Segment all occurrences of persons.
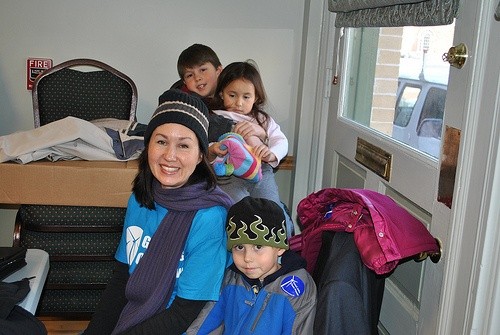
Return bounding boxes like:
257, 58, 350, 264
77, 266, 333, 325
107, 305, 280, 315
207, 59, 294, 239
182, 196, 317, 335
82, 89, 235, 335
170, 44, 223, 106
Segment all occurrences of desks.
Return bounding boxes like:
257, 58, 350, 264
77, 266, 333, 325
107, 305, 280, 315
0, 247, 49, 335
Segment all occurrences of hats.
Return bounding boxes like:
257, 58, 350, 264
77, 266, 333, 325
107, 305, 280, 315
225, 196, 289, 251
213, 132, 263, 184
144, 89, 210, 155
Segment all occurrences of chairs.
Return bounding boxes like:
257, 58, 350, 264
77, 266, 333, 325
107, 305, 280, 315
13, 59, 140, 321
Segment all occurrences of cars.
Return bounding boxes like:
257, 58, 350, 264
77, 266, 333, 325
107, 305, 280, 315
393, 75, 449, 159
